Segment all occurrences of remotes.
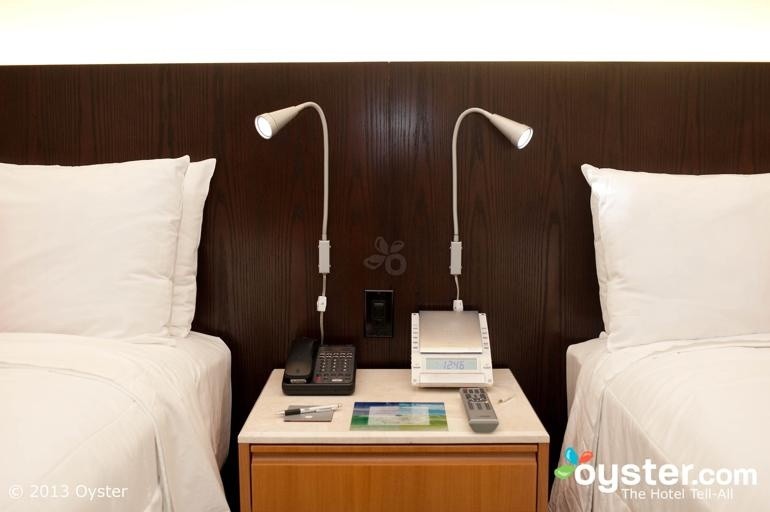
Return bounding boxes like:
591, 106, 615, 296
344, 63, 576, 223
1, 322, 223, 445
460, 387, 500, 433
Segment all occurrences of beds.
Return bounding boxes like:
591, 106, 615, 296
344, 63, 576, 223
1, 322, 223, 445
566, 338, 770, 512
1, 331, 232, 512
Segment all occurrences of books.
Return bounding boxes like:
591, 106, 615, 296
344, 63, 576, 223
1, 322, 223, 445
347, 400, 449, 434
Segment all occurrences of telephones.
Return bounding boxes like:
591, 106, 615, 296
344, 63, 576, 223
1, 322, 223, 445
282, 335, 357, 396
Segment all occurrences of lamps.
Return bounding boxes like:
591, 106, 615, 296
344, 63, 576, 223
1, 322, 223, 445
252, 101, 329, 344
450, 108, 533, 314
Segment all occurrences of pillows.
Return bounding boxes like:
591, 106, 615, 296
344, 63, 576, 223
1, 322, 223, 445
581, 164, 770, 339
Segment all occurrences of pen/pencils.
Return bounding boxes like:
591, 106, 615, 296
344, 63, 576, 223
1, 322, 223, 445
276, 404, 339, 415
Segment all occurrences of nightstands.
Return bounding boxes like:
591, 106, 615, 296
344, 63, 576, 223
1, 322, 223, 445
236, 367, 553, 511
